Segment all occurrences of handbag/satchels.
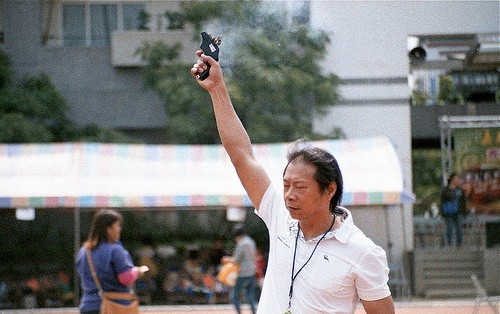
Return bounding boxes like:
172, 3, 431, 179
216, 262, 239, 287
442, 201, 458, 215
98, 291, 139, 314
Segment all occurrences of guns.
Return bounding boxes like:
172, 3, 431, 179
196, 31, 221, 81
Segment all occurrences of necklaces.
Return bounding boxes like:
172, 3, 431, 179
285, 214, 336, 314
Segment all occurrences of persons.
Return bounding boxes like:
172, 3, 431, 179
441, 174, 467, 246
137, 228, 264, 314
77, 211, 144, 314
191, 51, 395, 314
